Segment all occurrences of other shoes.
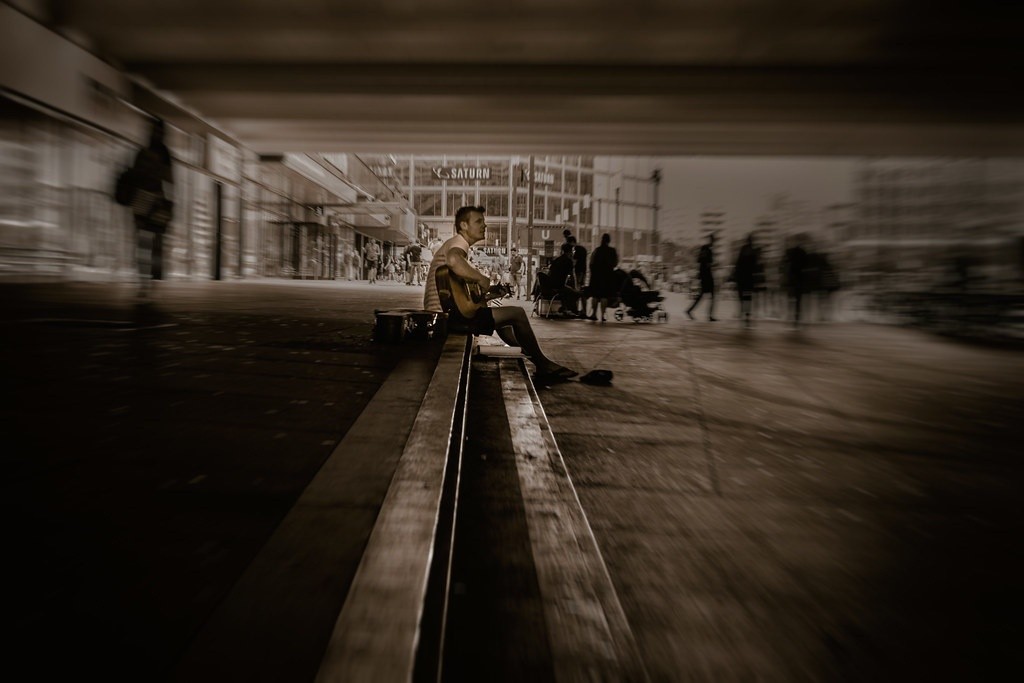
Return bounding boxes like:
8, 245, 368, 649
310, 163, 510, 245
565, 310, 588, 320
602, 317, 606, 321
686, 311, 693, 319
710, 317, 718, 321
587, 315, 598, 321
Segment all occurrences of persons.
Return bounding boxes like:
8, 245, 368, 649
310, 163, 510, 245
424, 206, 579, 383
341, 230, 818, 331
128, 119, 174, 293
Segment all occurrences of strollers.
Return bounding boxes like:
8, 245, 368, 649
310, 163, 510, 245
614, 268, 670, 325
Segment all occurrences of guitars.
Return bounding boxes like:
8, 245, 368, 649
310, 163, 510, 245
435, 263, 514, 322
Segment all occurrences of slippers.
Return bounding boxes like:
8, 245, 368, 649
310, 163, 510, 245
533, 367, 578, 382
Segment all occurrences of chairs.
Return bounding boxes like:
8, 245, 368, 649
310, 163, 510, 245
531, 272, 568, 319
